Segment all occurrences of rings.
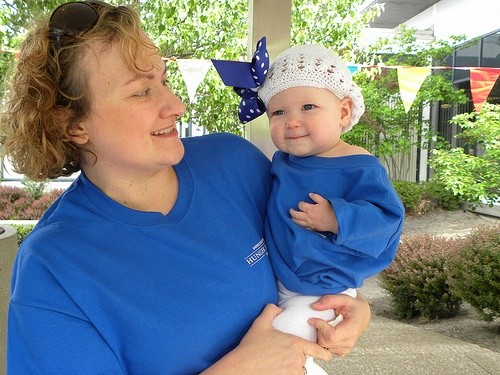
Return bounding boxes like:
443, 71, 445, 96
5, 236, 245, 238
302, 366, 307, 375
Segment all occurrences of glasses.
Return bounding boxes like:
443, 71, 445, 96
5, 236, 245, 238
46, 1, 115, 76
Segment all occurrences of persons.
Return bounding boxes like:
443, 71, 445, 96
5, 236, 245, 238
210, 37, 406, 375
0, 0, 371, 375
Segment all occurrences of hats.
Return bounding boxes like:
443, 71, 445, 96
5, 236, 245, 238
211, 37, 366, 134
